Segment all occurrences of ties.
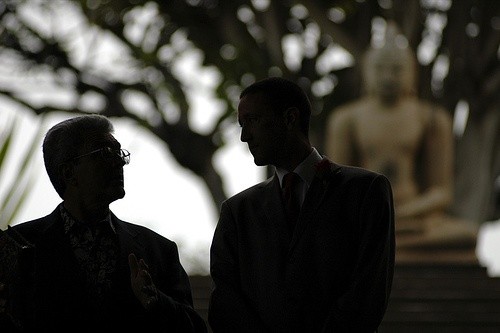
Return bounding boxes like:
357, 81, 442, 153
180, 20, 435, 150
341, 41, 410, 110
283, 173, 300, 232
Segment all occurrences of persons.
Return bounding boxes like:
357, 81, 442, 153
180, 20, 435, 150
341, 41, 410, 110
208, 76, 396, 333
327, 43, 478, 253
0, 116, 208, 333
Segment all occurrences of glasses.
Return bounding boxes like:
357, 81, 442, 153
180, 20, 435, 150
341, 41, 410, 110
70, 139, 129, 162
